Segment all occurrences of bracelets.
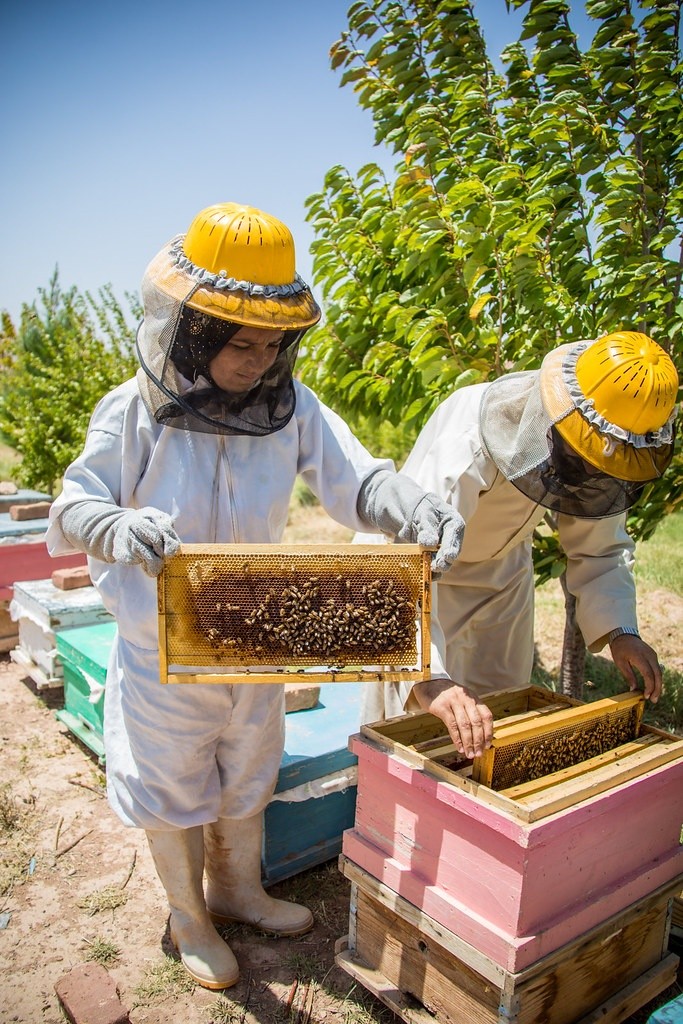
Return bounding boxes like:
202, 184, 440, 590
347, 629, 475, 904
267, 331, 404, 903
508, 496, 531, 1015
606, 627, 642, 646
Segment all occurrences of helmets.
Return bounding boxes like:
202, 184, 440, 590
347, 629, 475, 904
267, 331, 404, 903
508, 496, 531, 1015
144, 202, 321, 339
546, 331, 679, 481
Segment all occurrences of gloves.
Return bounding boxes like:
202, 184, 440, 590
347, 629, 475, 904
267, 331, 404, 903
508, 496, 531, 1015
361, 468, 466, 581
68, 501, 183, 577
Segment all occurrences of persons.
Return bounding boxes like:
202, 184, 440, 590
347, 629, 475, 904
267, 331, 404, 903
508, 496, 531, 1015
44, 202, 464, 989
345, 328, 679, 760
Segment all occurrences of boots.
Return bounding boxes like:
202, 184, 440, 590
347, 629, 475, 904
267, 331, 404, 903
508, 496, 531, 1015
147, 826, 241, 991
200, 817, 316, 937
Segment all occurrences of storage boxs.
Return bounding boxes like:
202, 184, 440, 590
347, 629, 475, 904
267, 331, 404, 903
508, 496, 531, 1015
0, 490, 359, 888
334, 852, 681, 1024
342, 681, 683, 973
